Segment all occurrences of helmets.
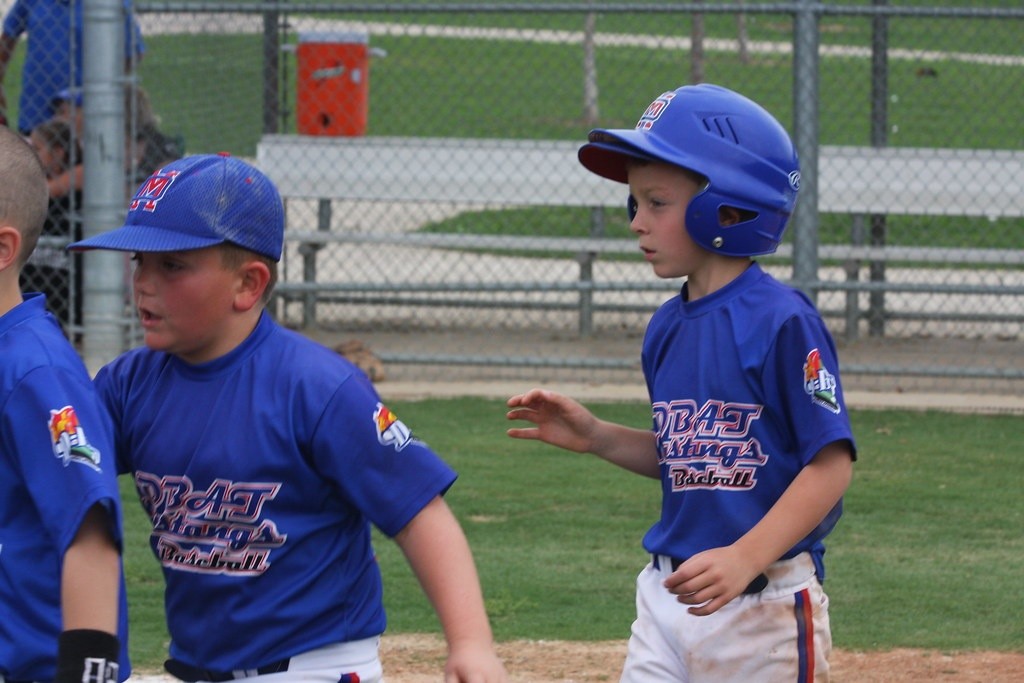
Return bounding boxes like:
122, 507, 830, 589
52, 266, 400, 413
587, 83, 802, 255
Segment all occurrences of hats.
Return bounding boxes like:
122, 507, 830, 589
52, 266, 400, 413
66, 152, 286, 261
575, 140, 661, 182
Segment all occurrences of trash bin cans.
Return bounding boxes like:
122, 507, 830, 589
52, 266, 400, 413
281, 21, 386, 137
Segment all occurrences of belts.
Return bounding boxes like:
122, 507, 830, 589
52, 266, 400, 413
652, 554, 769, 595
162, 657, 288, 682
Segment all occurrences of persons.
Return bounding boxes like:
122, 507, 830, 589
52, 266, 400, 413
0, 125, 131, 683
508, 83, 858, 683
66, 154, 509, 683
0, 0, 185, 343
